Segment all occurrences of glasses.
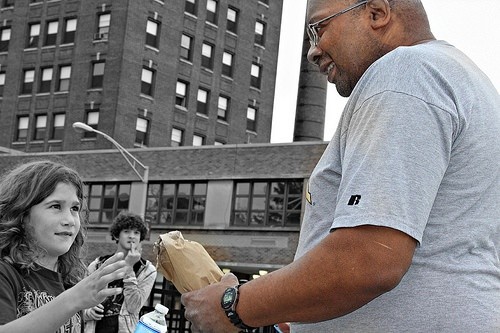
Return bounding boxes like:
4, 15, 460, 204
306, 0, 368, 47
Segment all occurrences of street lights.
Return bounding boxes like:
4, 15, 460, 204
71, 121, 149, 221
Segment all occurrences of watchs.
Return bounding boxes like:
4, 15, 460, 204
221, 284, 256, 329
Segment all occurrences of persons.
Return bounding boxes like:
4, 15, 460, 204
0, 161, 126, 333
83, 211, 157, 333
181, 0, 500, 333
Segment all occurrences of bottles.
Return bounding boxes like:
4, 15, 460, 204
134, 303, 169, 333
